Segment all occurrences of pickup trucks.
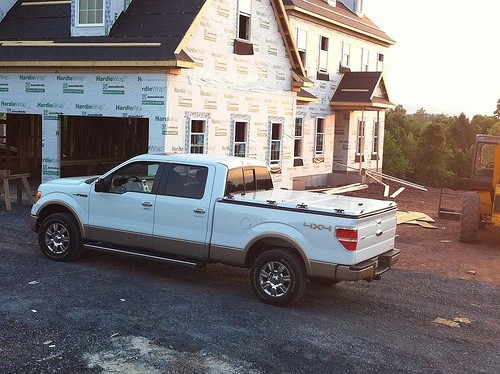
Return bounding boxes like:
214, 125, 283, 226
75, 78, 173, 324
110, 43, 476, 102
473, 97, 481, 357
30, 154, 401, 307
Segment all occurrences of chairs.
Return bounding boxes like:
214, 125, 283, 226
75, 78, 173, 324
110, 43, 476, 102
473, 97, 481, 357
194, 170, 208, 187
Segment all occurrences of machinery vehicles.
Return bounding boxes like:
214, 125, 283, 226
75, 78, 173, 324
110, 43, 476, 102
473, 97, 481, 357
458, 134, 500, 244
